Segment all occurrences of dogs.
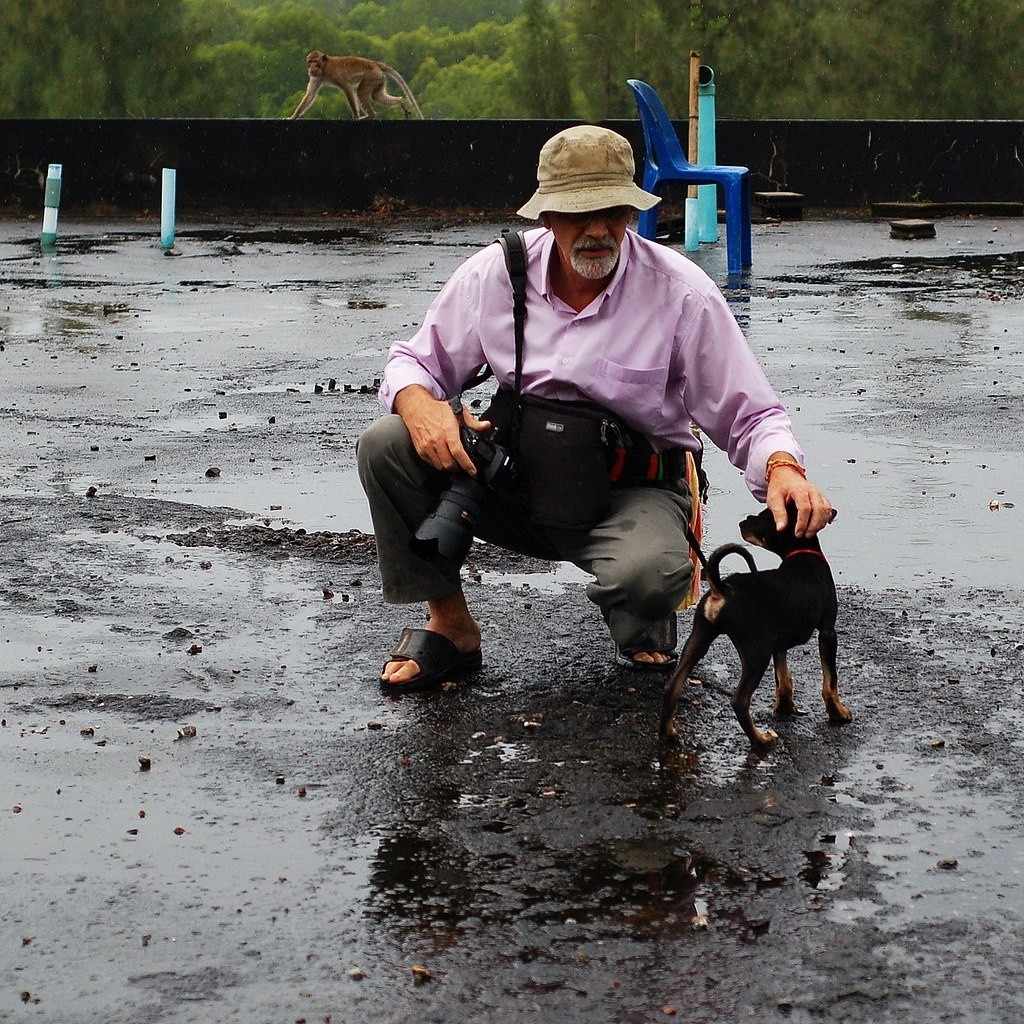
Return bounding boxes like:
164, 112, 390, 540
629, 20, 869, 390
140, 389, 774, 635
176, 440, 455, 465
659, 500, 852, 758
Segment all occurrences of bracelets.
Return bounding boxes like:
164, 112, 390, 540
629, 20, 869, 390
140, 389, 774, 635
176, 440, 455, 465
766, 459, 806, 483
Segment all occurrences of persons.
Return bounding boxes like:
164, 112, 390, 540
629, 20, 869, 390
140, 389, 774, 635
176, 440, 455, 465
355, 125, 832, 687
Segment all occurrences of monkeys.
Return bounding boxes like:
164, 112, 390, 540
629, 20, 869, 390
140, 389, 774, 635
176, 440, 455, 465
289, 51, 425, 120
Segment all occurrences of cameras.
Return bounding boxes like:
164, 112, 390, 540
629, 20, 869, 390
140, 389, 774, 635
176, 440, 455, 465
407, 424, 518, 577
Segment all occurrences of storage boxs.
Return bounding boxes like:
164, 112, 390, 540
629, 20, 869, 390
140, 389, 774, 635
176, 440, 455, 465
754, 191, 805, 222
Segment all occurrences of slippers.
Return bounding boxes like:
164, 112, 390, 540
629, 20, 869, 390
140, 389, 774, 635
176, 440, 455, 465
614, 641, 680, 668
380, 623, 484, 687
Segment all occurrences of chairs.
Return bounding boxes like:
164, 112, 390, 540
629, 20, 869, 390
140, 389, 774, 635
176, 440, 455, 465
626, 76, 755, 277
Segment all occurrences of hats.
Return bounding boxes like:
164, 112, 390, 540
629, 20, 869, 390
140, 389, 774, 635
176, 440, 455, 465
516, 124, 663, 220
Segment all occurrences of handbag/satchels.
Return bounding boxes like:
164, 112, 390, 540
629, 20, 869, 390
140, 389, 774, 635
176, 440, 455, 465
495, 393, 691, 486
